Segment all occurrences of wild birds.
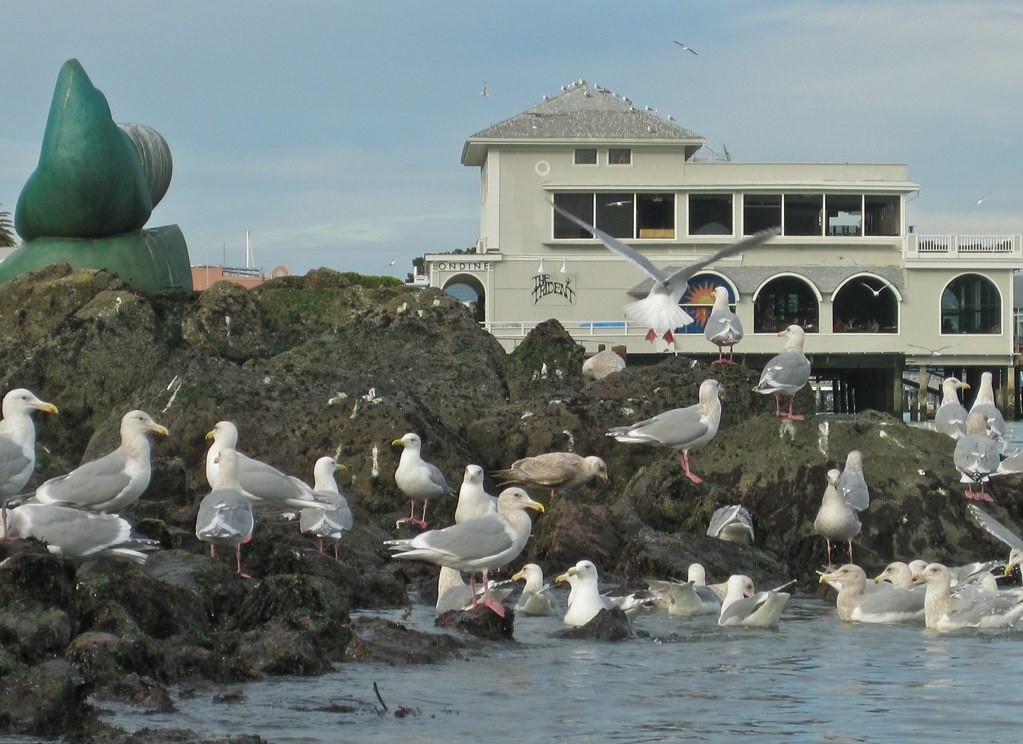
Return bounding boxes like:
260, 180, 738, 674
673, 40, 698, 55
480, 79, 490, 96
0, 195, 1021, 636
541, 78, 680, 131
